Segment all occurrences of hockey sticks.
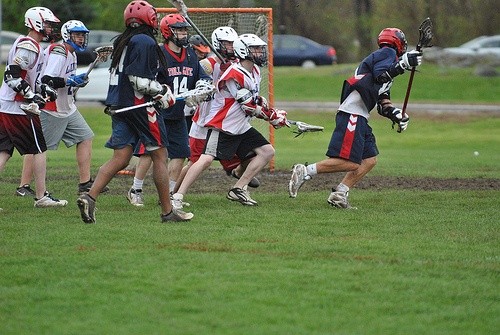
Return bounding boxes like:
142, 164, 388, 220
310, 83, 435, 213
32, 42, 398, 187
108, 80, 219, 115
255, 96, 326, 133
167, 0, 227, 64
19, 95, 52, 118
73, 45, 113, 96
396, 17, 433, 133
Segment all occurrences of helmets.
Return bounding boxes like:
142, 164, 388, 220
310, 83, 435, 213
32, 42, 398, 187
211, 26, 239, 60
25, 0, 190, 53
189, 34, 210, 60
233, 33, 269, 66
378, 28, 408, 57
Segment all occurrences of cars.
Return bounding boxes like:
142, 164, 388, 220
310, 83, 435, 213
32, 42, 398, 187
0, 29, 123, 101
261, 33, 338, 67
443, 32, 500, 66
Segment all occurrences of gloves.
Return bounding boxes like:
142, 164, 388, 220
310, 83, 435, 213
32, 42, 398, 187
40, 80, 58, 102
258, 95, 269, 110
151, 83, 175, 108
66, 74, 89, 87
238, 91, 256, 116
267, 108, 288, 130
387, 107, 409, 131
196, 80, 214, 101
24, 92, 46, 110
398, 49, 423, 69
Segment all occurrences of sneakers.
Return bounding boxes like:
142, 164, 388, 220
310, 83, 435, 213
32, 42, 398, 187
327, 188, 358, 211
77, 179, 109, 196
227, 186, 258, 207
126, 187, 144, 207
287, 161, 311, 197
76, 192, 96, 224
16, 185, 36, 196
170, 196, 182, 209
34, 191, 68, 208
231, 169, 260, 187
158, 192, 190, 206
162, 207, 194, 222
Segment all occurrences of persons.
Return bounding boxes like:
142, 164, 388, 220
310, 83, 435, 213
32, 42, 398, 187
160, 26, 261, 204
289, 28, 423, 208
128, 14, 215, 210
76, 0, 194, 223
13, 18, 110, 197
172, 32, 289, 221
183, 34, 211, 132
0, 7, 69, 208
